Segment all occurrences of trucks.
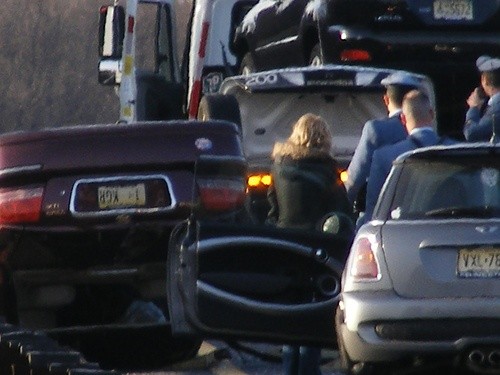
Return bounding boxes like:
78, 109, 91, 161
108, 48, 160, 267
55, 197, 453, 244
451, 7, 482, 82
93, 0, 239, 124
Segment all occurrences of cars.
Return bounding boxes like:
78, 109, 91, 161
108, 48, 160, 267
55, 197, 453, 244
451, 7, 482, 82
332, 141, 500, 375
2, 120, 340, 375
228, 0, 499, 116
197, 63, 440, 225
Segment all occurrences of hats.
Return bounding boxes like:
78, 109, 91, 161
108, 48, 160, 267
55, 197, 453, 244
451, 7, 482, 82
477, 55, 500, 71
381, 70, 424, 87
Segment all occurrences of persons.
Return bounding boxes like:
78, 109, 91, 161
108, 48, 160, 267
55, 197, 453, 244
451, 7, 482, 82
462, 54, 500, 144
343, 69, 469, 236
266, 112, 356, 375
